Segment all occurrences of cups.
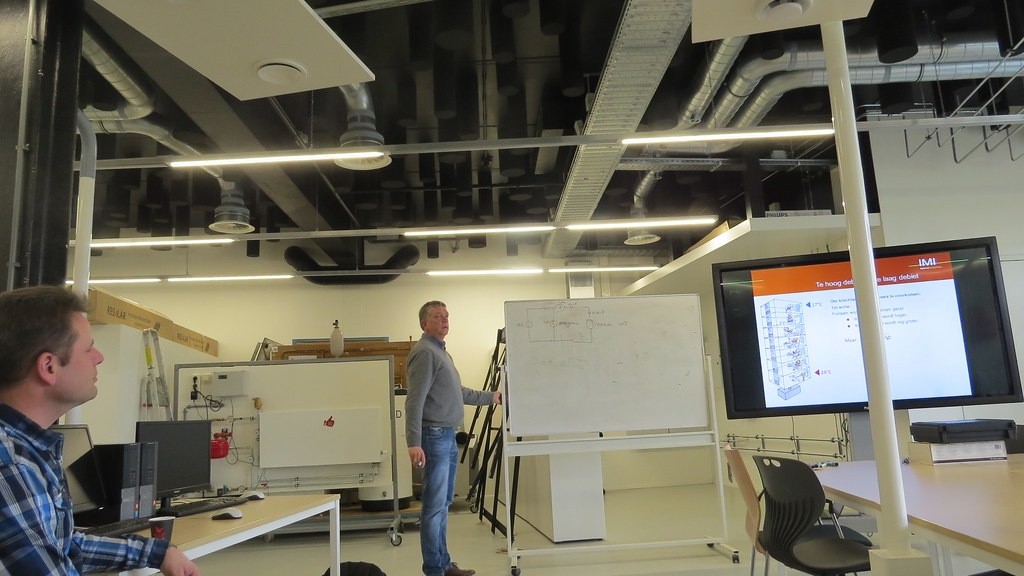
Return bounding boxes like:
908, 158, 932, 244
148, 516, 175, 542
217, 489, 223, 496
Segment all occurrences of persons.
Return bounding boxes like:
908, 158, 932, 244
0, 284, 200, 576
403, 300, 507, 576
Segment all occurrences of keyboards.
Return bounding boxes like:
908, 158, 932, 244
155, 497, 249, 516
80, 516, 157, 538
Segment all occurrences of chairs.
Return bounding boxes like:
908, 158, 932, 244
749, 454, 871, 576
725, 444, 874, 576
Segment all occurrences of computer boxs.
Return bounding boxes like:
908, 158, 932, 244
78, 443, 158, 526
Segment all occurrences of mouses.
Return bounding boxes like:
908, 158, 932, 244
212, 508, 243, 520
239, 490, 265, 500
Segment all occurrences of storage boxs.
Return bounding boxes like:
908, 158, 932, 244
910, 417, 1019, 442
906, 441, 1010, 465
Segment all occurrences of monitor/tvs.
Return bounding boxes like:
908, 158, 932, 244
47, 424, 108, 533
135, 420, 211, 510
711, 236, 1024, 420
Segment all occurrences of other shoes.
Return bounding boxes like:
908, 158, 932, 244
445, 562, 475, 576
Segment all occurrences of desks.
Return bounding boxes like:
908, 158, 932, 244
813, 453, 1024, 576
99, 493, 341, 576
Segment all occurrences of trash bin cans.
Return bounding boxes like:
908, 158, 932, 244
330, 558, 387, 575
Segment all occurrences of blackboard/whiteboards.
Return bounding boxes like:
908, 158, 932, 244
173, 358, 398, 494
501, 297, 715, 445
